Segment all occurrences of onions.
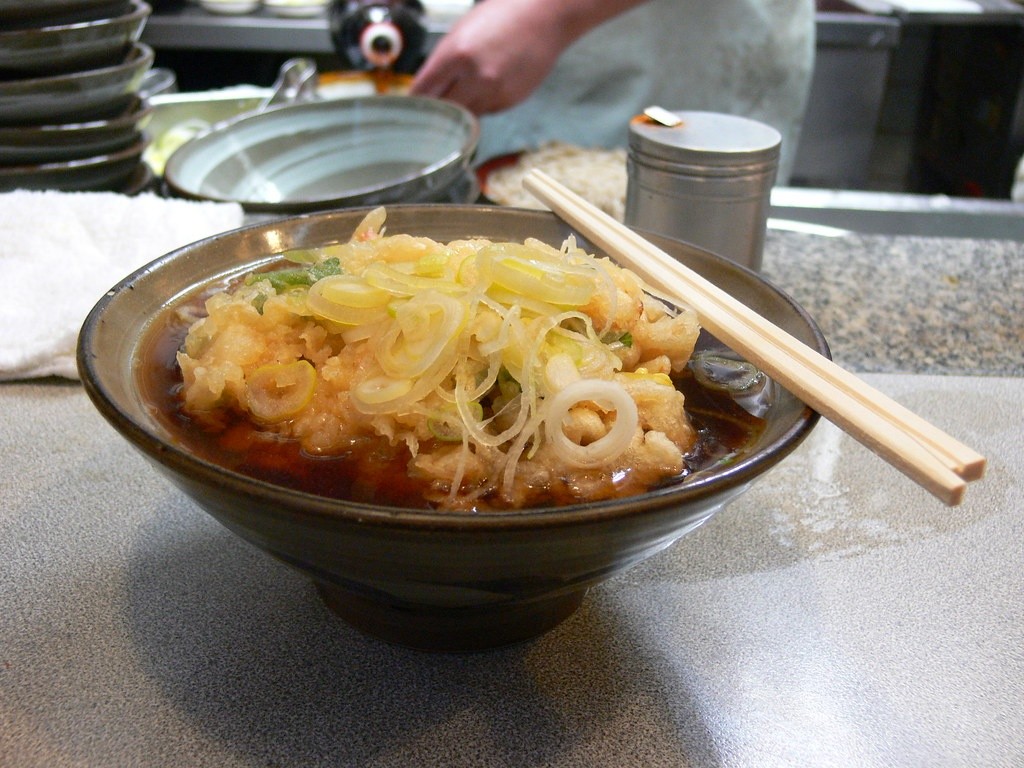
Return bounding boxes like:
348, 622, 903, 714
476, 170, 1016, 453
225, 239, 637, 497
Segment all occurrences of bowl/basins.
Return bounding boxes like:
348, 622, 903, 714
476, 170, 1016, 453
475, 150, 537, 207
76, 205, 832, 648
163, 91, 479, 213
0, 0, 154, 197
197, 0, 263, 14
263, 0, 332, 17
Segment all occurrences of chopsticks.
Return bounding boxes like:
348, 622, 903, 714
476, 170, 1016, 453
523, 169, 985, 507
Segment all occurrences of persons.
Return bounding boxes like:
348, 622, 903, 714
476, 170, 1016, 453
408, 0, 816, 188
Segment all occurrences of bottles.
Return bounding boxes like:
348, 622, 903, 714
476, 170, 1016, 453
625, 104, 782, 275
